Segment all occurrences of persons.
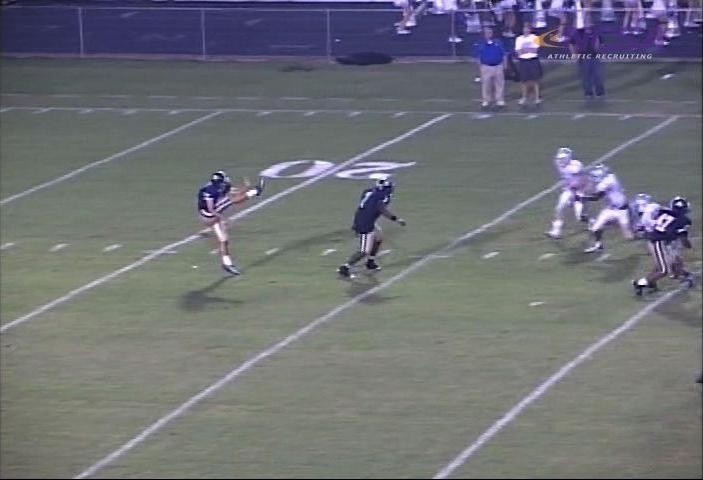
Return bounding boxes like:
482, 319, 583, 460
393, 0, 703, 110
197, 170, 265, 277
335, 178, 408, 279
544, 147, 696, 298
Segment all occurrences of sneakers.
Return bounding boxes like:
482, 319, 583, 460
544, 229, 563, 238
366, 264, 382, 272
219, 264, 241, 275
335, 263, 358, 280
254, 175, 266, 196
688, 272, 697, 289
630, 279, 643, 297
481, 99, 543, 106
582, 240, 606, 253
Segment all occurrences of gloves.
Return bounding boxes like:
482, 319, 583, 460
391, 215, 409, 228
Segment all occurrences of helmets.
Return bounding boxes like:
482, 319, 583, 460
588, 162, 608, 183
668, 195, 691, 214
633, 193, 651, 214
374, 177, 396, 194
211, 171, 225, 187
552, 146, 572, 168
